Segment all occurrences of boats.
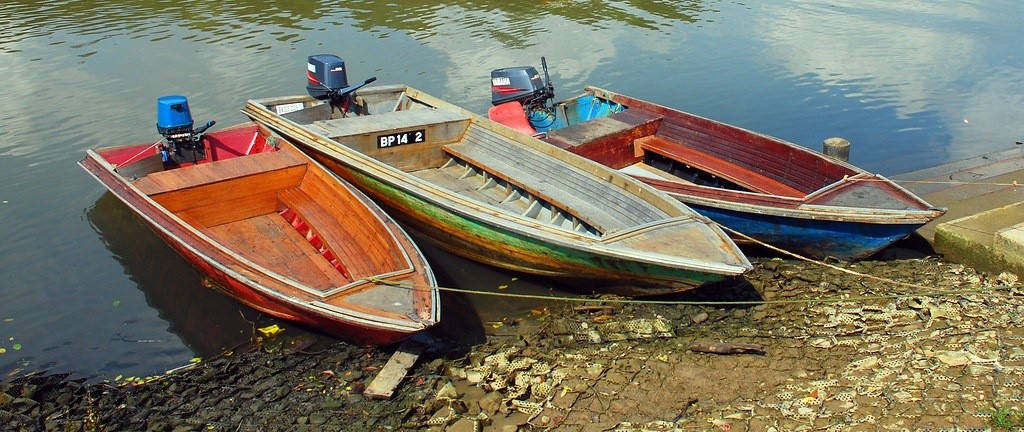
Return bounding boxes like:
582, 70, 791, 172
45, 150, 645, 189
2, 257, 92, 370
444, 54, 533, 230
486, 56, 951, 266
77, 95, 442, 350
239, 53, 754, 299
